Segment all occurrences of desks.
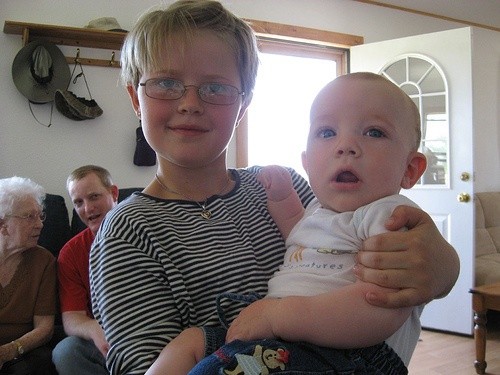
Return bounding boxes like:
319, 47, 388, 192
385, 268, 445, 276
467, 281, 500, 375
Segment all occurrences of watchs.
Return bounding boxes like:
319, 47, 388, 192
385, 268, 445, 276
15, 340, 24, 354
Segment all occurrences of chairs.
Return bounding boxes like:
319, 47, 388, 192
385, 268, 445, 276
32, 186, 146, 375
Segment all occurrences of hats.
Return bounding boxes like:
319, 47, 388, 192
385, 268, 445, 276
11, 40, 72, 104
54, 87, 103, 121
82, 16, 130, 34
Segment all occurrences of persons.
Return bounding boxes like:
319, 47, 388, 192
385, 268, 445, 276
88, 0, 461, 375
0, 176, 58, 375
144, 73, 427, 375
53, 165, 119, 375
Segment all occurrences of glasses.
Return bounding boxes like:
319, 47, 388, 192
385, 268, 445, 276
7, 211, 48, 224
138, 77, 249, 106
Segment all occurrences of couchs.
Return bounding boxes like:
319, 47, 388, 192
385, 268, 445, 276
473, 191, 500, 287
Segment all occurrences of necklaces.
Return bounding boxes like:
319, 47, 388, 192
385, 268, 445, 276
155, 175, 212, 220
